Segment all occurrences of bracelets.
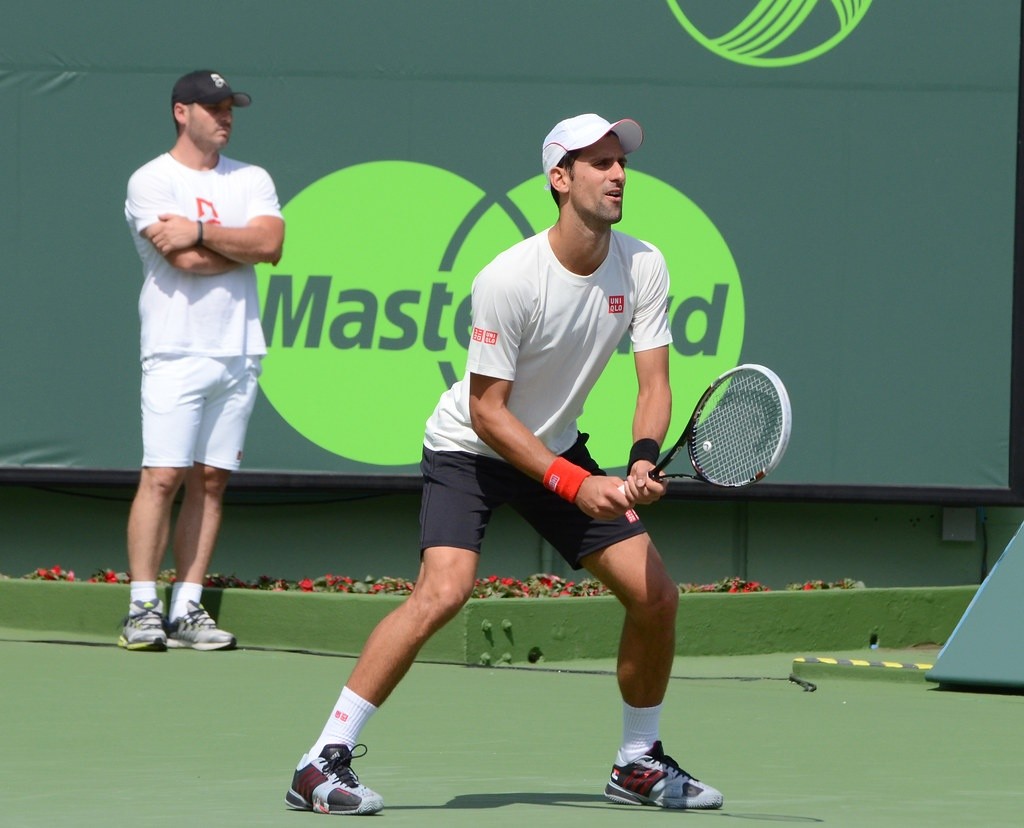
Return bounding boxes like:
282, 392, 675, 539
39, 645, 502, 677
627, 439, 660, 475
542, 456, 591, 503
195, 220, 203, 247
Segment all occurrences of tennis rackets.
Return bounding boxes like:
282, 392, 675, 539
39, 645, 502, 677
616, 363, 794, 497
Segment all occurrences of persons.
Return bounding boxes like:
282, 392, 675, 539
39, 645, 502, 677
118, 70, 285, 652
284, 113, 723, 817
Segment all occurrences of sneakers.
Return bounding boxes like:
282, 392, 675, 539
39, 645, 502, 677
162, 600, 236, 651
603, 741, 724, 810
284, 744, 384, 816
118, 598, 168, 651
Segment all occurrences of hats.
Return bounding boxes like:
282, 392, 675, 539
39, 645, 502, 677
171, 70, 252, 108
542, 112, 644, 183
